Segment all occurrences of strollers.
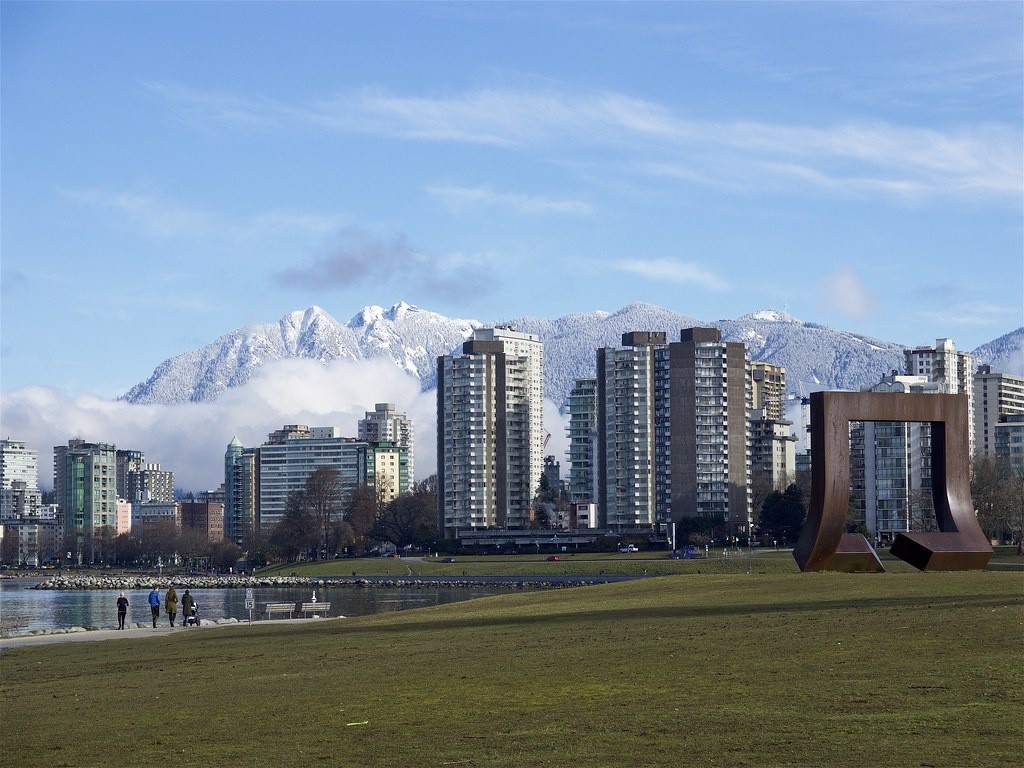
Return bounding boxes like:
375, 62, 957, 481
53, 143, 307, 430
187, 602, 200, 626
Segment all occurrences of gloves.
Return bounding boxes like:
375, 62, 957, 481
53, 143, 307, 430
124, 603, 127, 606
118, 604, 120, 606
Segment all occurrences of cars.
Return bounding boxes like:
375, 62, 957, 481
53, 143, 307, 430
382, 550, 395, 557
1, 564, 56, 570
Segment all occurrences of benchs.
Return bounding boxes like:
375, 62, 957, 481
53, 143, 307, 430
0, 615, 30, 639
260, 603, 296, 621
298, 601, 332, 618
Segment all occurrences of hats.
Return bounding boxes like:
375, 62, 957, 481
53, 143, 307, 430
120, 592, 124, 595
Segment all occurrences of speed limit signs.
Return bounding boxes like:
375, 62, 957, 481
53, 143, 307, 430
244, 599, 254, 610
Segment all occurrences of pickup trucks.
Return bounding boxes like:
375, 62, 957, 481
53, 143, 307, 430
620, 545, 638, 553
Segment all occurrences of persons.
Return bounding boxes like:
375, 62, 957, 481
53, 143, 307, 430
185, 566, 257, 578
182, 590, 195, 627
116, 591, 130, 630
165, 586, 178, 627
866, 536, 894, 549
272, 570, 300, 587
148, 586, 160, 628
672, 546, 694, 560
751, 535, 787, 552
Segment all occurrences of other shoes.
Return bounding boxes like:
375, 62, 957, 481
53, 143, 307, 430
170, 621, 174, 627
122, 627, 124, 630
118, 625, 121, 630
153, 626, 157, 628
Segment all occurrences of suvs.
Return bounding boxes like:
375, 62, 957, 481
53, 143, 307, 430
309, 551, 325, 561
546, 556, 559, 561
441, 558, 455, 563
